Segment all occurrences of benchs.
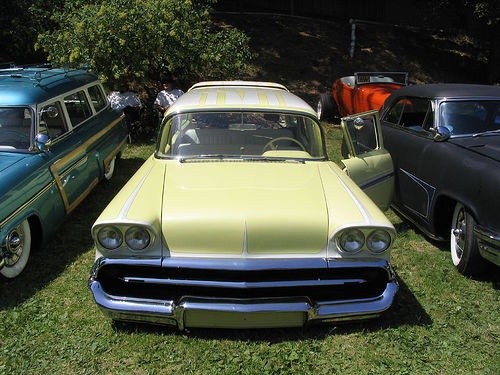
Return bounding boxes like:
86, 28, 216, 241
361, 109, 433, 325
394, 111, 489, 133
0, 116, 83, 129
183, 127, 293, 145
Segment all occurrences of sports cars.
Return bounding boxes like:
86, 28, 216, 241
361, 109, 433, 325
316, 71, 417, 122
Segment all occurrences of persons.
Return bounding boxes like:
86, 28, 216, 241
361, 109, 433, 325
106, 82, 142, 123
152, 77, 184, 111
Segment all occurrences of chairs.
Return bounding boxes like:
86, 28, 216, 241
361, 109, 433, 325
341, 75, 393, 88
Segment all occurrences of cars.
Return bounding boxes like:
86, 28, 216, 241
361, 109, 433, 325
339, 82, 500, 279
88, 79, 400, 332
0, 65, 128, 292
